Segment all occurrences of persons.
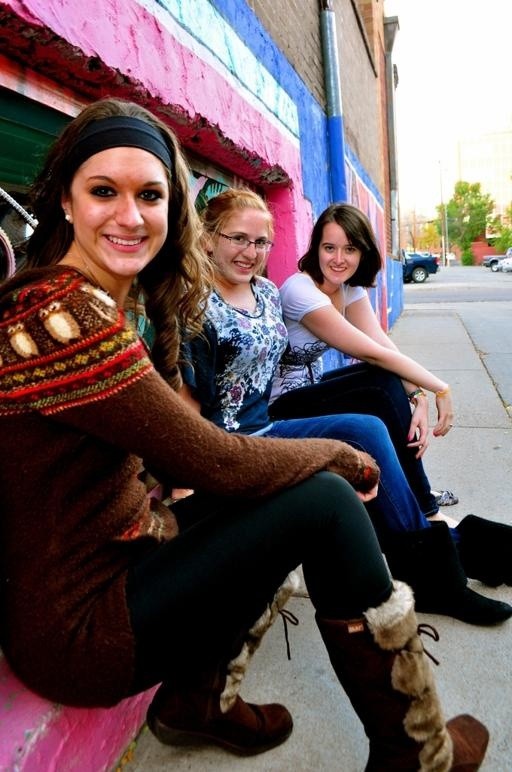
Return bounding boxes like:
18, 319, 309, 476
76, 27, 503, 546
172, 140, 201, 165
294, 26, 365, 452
0, 99, 490, 772
274, 202, 463, 531
174, 185, 511, 628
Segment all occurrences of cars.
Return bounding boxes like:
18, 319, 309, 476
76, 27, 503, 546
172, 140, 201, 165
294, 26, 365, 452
402, 248, 439, 284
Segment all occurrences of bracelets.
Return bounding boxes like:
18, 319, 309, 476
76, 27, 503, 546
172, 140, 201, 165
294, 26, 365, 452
436, 385, 451, 398
408, 389, 425, 402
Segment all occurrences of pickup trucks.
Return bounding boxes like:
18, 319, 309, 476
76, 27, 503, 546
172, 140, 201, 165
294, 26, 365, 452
483, 247, 512, 273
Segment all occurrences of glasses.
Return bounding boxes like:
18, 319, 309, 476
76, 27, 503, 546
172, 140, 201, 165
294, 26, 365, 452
216, 231, 274, 254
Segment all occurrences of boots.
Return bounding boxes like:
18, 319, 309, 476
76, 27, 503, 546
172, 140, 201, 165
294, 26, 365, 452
385, 520, 512, 628
147, 568, 294, 757
313, 580, 490, 771
456, 513, 510, 590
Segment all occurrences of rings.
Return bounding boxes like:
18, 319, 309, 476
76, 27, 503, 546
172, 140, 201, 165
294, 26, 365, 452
449, 424, 454, 428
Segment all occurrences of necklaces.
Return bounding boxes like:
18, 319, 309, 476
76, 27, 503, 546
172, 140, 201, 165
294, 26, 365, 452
73, 241, 100, 287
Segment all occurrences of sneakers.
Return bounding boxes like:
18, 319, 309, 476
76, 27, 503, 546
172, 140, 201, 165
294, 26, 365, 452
433, 489, 458, 506
